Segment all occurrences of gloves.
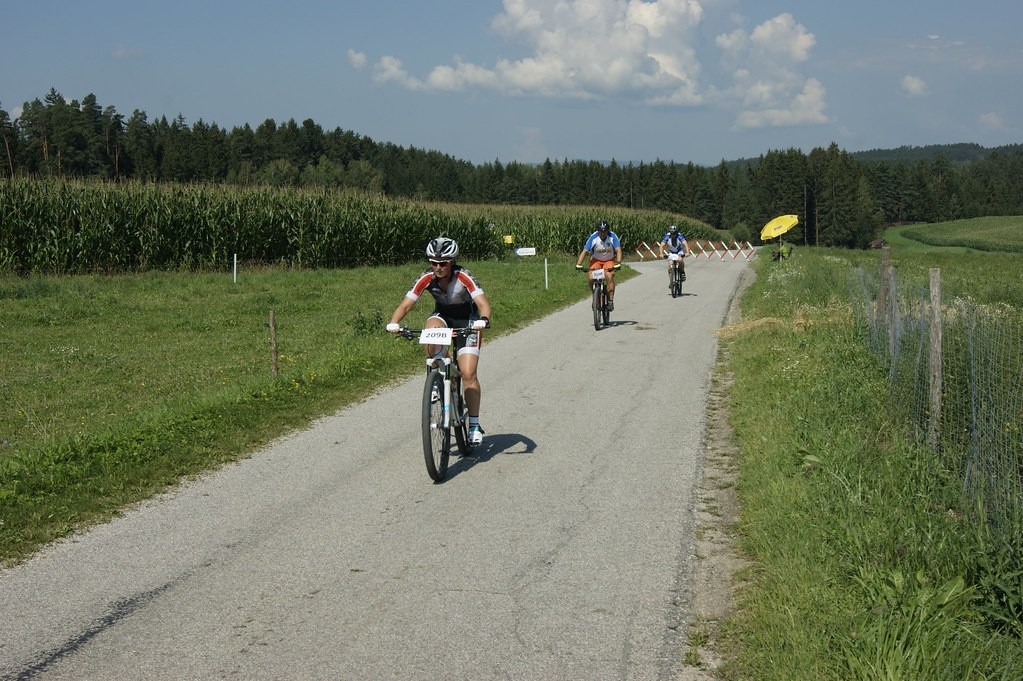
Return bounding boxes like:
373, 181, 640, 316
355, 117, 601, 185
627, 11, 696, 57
613, 261, 621, 270
576, 264, 583, 270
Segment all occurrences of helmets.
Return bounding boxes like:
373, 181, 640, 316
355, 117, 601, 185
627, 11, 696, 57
669, 225, 678, 233
596, 220, 609, 232
426, 237, 459, 258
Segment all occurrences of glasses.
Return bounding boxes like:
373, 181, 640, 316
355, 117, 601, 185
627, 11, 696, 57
670, 233, 677, 235
429, 259, 453, 267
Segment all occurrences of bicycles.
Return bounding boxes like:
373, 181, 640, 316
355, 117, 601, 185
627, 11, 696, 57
386, 324, 490, 483
573, 263, 622, 330
660, 253, 687, 298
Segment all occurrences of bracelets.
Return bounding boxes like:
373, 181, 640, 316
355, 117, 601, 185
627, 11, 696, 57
480, 317, 490, 325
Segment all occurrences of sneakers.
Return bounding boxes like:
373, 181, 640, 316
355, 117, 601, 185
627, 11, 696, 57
430, 381, 442, 404
467, 425, 484, 447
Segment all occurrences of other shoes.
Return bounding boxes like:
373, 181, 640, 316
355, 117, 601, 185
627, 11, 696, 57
606, 304, 614, 312
592, 303, 599, 311
680, 272, 686, 281
669, 283, 674, 289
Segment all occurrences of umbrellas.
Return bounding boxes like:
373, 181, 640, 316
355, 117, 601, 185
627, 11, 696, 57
761, 215, 798, 260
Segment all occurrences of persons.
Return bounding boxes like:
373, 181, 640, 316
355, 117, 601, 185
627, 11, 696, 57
577, 221, 622, 311
387, 237, 491, 447
772, 241, 788, 262
660, 225, 690, 288
